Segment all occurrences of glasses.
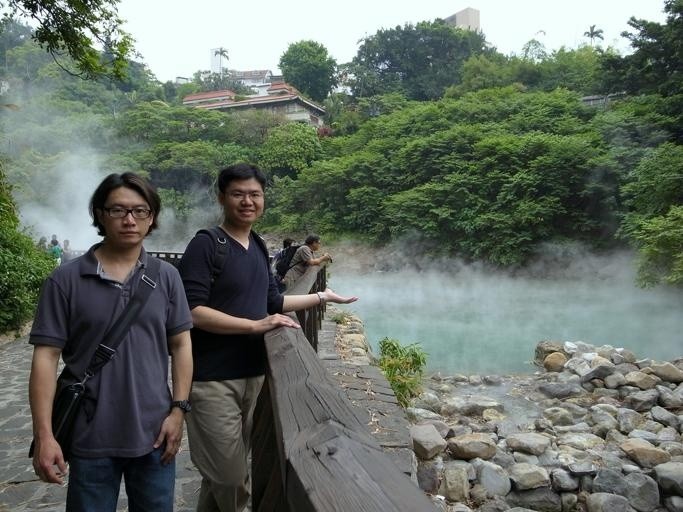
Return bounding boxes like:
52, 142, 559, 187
230, 191, 264, 201
100, 205, 154, 219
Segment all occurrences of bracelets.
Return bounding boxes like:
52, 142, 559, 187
317, 292, 324, 303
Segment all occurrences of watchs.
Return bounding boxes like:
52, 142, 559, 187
171, 400, 192, 413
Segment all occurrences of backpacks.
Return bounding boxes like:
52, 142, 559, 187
270, 243, 314, 282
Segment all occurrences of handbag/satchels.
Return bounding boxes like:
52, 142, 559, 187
28, 365, 85, 458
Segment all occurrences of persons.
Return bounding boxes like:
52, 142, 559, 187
167, 164, 358, 512
271, 235, 332, 294
40, 235, 69, 266
29, 172, 193, 512
